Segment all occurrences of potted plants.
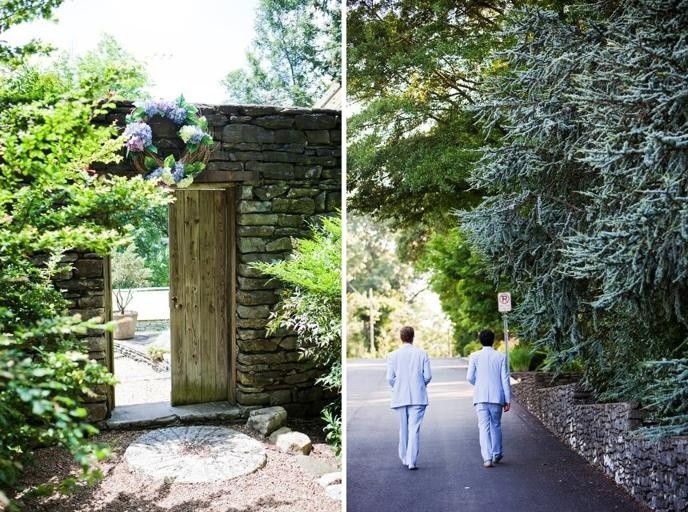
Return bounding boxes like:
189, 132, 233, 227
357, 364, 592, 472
109, 243, 153, 340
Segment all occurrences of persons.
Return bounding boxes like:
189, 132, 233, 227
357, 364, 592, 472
387, 327, 433, 470
466, 329, 513, 467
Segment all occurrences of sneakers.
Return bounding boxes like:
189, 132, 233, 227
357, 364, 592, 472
484, 455, 503, 466
409, 464, 416, 469
